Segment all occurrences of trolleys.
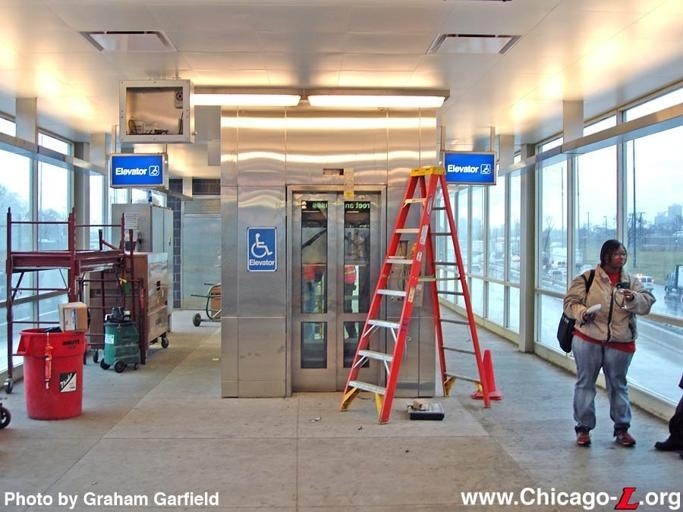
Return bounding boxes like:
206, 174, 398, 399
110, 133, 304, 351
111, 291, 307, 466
76, 277, 170, 363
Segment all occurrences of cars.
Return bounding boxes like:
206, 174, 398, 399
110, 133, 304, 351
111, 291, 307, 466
471, 236, 683, 318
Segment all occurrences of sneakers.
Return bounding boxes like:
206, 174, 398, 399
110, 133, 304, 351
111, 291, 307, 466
575, 430, 591, 445
654, 433, 681, 451
615, 431, 636, 446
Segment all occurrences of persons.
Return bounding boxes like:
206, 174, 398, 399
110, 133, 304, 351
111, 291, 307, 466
651, 372, 683, 459
318, 255, 358, 341
560, 239, 656, 448
300, 263, 326, 341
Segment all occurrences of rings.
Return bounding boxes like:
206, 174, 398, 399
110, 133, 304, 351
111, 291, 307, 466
626, 295, 629, 298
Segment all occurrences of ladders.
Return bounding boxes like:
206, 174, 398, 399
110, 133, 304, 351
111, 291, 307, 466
339, 165, 490, 426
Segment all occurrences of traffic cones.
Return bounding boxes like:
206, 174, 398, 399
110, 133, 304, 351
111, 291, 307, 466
471, 347, 502, 401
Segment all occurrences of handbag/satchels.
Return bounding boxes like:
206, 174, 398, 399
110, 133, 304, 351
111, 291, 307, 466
556, 312, 576, 354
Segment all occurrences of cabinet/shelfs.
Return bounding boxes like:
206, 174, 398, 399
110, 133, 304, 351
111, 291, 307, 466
111, 203, 174, 316
88, 251, 170, 351
